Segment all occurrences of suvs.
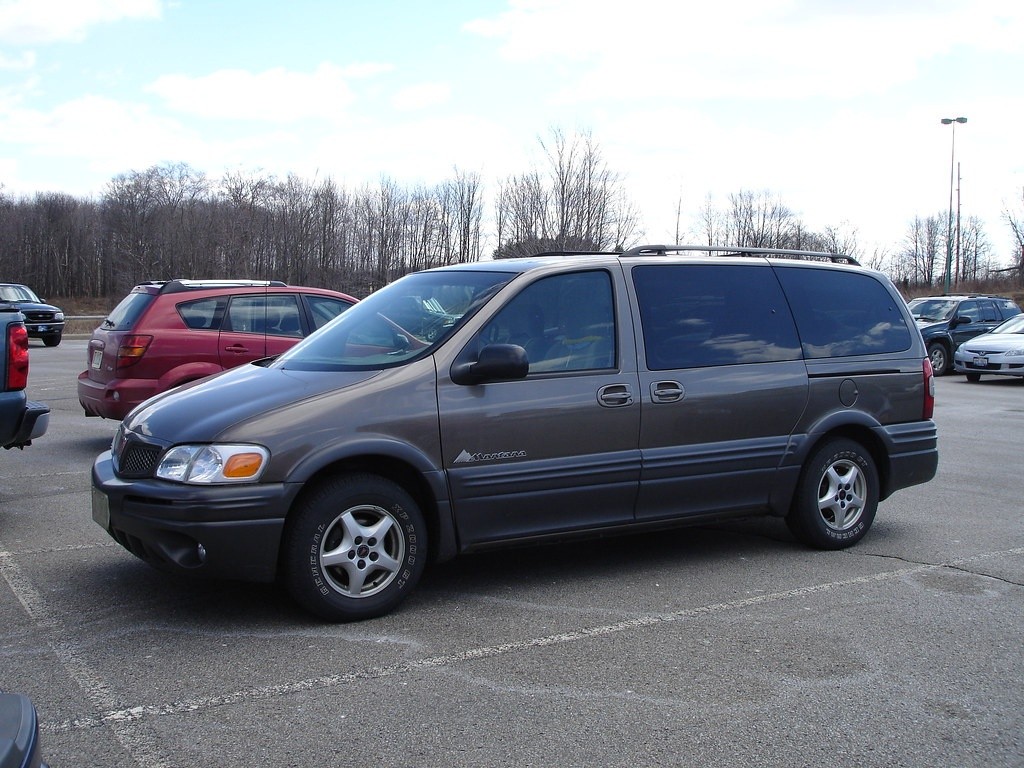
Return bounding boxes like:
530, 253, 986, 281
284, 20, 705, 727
1, 283, 64, 348
907, 294, 1024, 383
95, 247, 940, 621
77, 280, 436, 422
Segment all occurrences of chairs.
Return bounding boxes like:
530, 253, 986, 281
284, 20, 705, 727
498, 303, 547, 365
257, 307, 301, 337
186, 310, 206, 328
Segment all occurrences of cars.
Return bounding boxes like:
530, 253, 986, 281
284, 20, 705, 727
1, 313, 51, 450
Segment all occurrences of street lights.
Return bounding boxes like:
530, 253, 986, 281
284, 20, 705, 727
943, 116, 970, 287
